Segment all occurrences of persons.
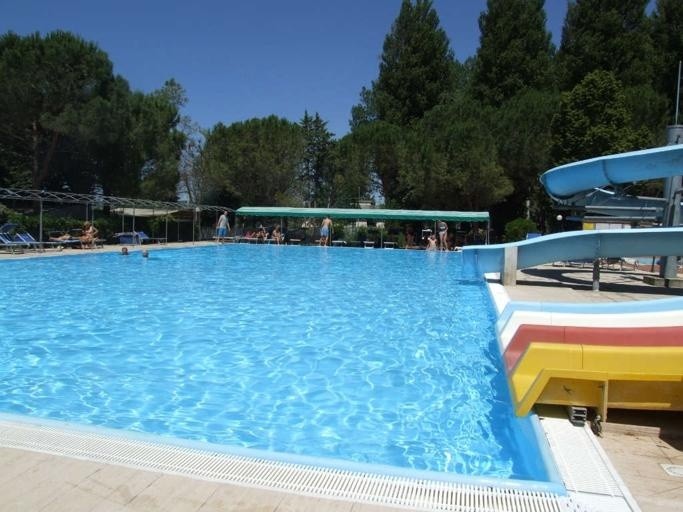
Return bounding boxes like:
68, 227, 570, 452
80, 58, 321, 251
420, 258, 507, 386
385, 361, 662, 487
216, 210, 230, 244
48, 234, 80, 241
427, 235, 437, 251
271, 223, 282, 247
437, 220, 448, 252
141, 250, 148, 257
255, 230, 269, 238
79, 221, 97, 249
121, 247, 128, 255
244, 230, 255, 238
318, 215, 334, 247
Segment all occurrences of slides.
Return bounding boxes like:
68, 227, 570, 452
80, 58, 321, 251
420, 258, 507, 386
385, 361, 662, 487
496, 297, 683, 417
461, 144, 683, 273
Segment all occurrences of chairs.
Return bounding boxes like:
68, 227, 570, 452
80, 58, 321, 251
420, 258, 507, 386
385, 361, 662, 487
0, 229, 107, 254
212, 233, 400, 248
136, 231, 167, 245
